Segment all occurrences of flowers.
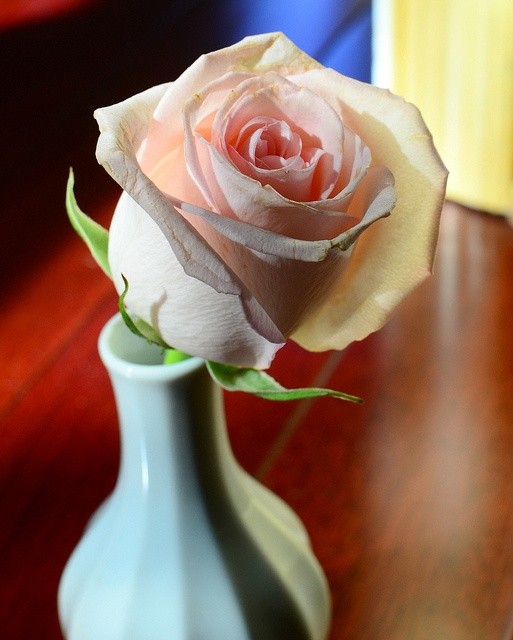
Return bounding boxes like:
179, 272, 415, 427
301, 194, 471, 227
65, 30, 450, 403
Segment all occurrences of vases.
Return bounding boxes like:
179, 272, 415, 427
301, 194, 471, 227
55, 311, 331, 640
